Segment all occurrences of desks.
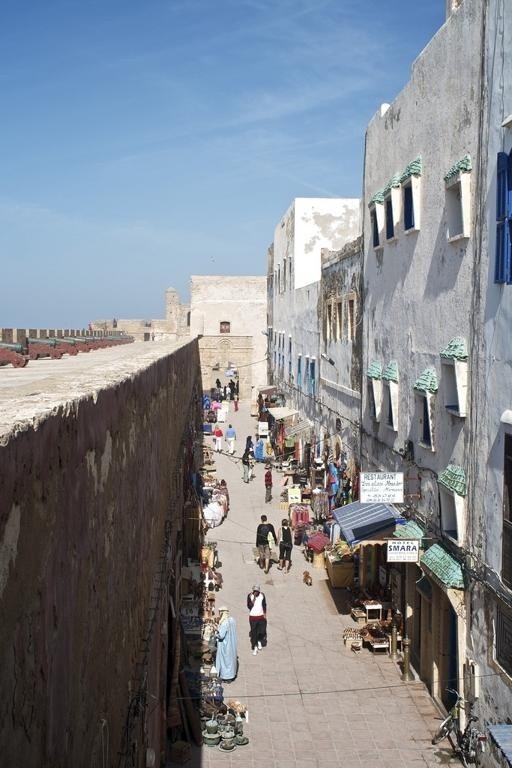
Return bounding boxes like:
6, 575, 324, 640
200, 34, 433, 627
351, 594, 405, 656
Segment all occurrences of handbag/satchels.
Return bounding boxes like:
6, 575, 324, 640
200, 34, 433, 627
253, 548, 260, 563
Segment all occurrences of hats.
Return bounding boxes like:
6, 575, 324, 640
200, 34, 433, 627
252, 585, 261, 593
218, 604, 231, 614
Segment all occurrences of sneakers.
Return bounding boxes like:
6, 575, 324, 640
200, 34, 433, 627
259, 561, 288, 574
252, 641, 263, 656
216, 450, 274, 502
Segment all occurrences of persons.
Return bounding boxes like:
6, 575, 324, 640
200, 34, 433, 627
277, 519, 293, 574
247, 584, 267, 654
256, 515, 277, 572
211, 606, 238, 683
212, 377, 274, 502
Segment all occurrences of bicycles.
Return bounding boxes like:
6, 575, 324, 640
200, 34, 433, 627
431, 689, 488, 767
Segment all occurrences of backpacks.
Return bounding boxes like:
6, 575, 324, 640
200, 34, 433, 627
282, 526, 292, 550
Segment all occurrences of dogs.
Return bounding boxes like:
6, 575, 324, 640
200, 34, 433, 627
302, 570, 312, 586
